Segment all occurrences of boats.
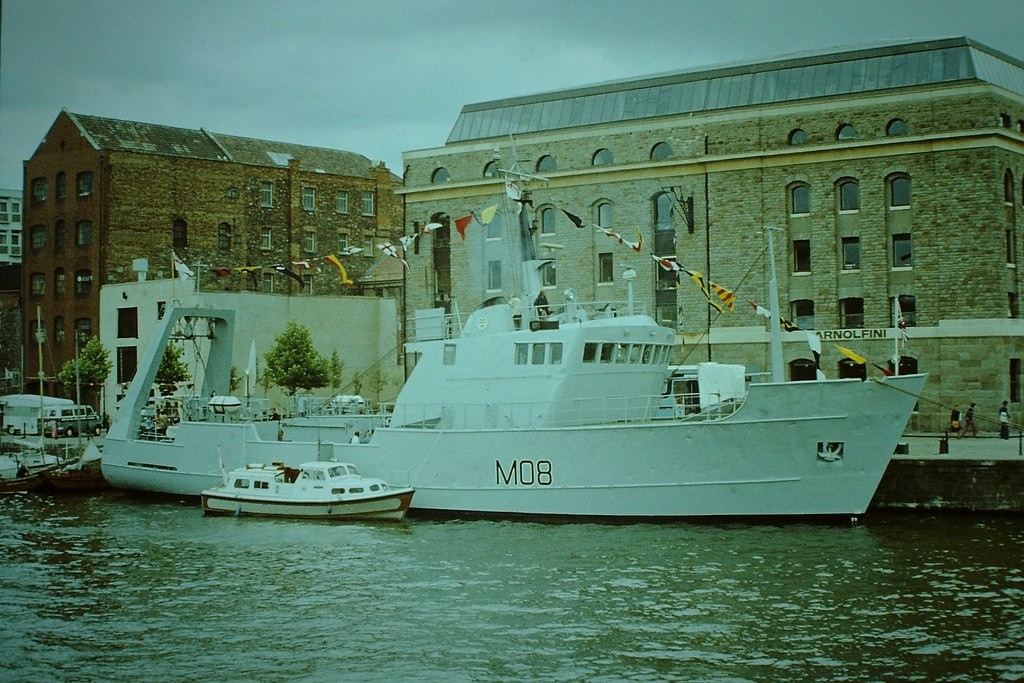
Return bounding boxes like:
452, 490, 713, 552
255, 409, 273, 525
203, 446, 413, 524
0, 451, 64, 496
103, 130, 928, 514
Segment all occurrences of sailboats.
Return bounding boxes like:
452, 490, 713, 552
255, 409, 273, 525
32, 306, 107, 494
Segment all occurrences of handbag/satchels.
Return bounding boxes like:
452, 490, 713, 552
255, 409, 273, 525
951, 420, 959, 428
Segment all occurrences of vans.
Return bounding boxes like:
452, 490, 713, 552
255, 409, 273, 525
40, 404, 105, 438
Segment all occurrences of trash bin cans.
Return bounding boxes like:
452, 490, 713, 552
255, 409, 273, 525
939, 430, 950, 454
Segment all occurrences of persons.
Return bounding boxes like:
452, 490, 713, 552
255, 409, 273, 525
351, 430, 374, 444
146, 409, 168, 441
998, 401, 1012, 441
961, 402, 979, 439
948, 404, 962, 440
16, 463, 29, 478
533, 289, 555, 323
509, 294, 525, 330
269, 408, 279, 421
105, 414, 110, 434
